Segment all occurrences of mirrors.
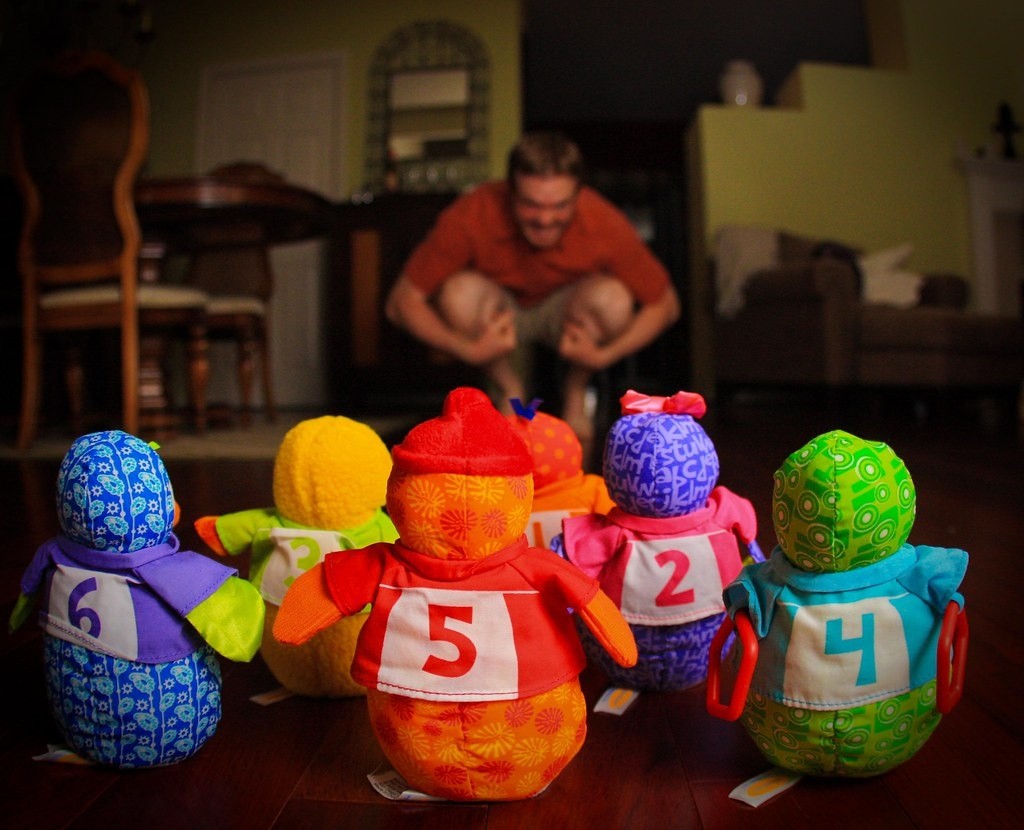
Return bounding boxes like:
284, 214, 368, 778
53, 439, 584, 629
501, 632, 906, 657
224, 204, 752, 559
359, 15, 492, 194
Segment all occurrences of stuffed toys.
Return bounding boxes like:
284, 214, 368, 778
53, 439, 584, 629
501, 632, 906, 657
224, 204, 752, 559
194, 416, 400, 695
273, 387, 638, 799
705, 431, 969, 779
9, 431, 265, 767
548, 390, 767, 693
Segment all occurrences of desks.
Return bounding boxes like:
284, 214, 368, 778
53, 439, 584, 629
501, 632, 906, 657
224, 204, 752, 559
129, 174, 333, 256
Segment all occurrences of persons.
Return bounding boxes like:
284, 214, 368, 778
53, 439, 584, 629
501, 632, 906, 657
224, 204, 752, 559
385, 135, 679, 436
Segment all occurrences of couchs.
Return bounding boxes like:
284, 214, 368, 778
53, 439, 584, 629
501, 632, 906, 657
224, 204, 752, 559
708, 220, 1023, 437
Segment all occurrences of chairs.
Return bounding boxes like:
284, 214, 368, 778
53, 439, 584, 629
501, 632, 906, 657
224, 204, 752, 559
6, 38, 212, 449
158, 158, 280, 430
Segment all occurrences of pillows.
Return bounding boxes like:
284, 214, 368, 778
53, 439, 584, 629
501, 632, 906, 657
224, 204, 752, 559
810, 241, 927, 310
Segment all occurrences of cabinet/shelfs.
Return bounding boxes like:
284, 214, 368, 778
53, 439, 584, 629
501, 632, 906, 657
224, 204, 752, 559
323, 190, 485, 418
